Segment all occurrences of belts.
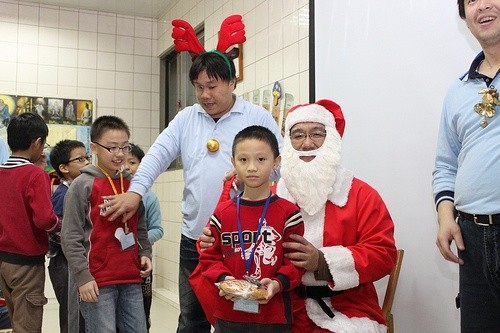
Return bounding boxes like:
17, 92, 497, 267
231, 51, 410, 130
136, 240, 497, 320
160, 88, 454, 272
459, 211, 500, 226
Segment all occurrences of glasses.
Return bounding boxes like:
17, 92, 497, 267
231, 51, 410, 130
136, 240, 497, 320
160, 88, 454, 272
65, 155, 91, 163
292, 132, 326, 140
93, 142, 132, 153
44, 143, 50, 148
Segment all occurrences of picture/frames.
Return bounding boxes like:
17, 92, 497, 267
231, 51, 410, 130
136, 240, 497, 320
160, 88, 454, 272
218, 31, 243, 82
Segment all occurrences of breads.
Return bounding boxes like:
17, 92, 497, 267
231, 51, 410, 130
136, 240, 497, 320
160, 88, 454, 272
219, 280, 268, 299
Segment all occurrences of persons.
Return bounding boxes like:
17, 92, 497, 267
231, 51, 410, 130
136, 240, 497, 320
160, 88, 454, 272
199, 125, 307, 333
46, 139, 92, 333
60, 115, 165, 333
188, 99, 397, 333
0, 112, 63, 333
98, 14, 283, 333
430, 0, 500, 333
